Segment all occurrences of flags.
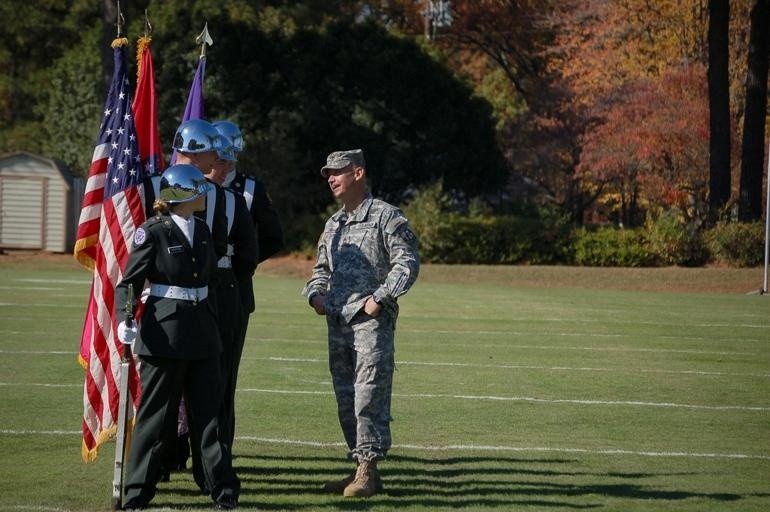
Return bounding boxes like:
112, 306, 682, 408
72, 37, 157, 471
167, 55, 216, 172
125, 31, 176, 212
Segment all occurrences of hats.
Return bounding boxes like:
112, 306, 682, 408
320, 148, 364, 178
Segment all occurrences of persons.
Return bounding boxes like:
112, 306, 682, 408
108, 162, 244, 511
200, 118, 273, 285
204, 134, 259, 452
158, 117, 268, 500
300, 146, 422, 503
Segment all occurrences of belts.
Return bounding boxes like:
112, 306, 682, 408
217, 253, 232, 270
149, 283, 208, 306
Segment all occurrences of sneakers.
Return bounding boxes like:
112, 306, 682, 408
218, 493, 240, 509
123, 498, 147, 511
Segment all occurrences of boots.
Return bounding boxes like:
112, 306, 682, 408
325, 459, 382, 498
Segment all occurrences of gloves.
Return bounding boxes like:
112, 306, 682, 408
117, 317, 138, 346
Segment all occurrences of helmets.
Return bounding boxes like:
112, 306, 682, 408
158, 165, 215, 202
171, 121, 244, 161
